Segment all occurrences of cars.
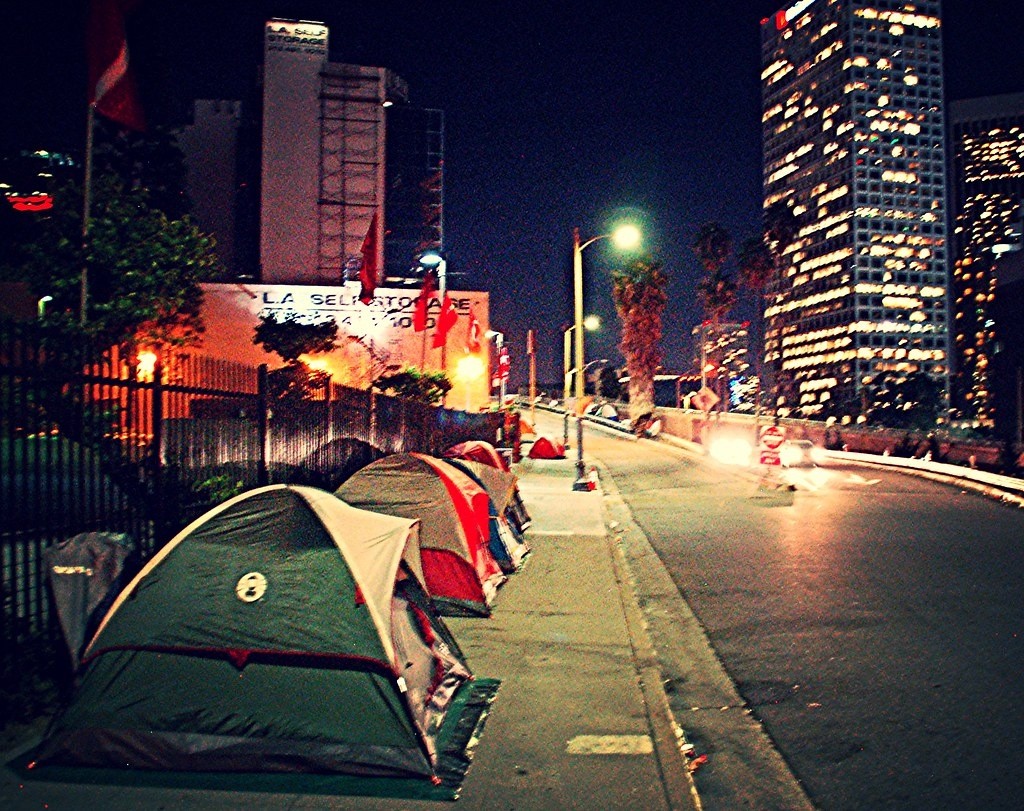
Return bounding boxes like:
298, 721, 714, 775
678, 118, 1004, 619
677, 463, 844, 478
779, 439, 818, 468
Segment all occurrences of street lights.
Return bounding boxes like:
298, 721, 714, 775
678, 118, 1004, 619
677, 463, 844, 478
563, 319, 597, 450
574, 228, 638, 493
421, 255, 448, 407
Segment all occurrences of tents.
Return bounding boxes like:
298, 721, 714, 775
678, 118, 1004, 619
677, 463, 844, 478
527, 430, 567, 460
16, 481, 498, 802
331, 438, 531, 619
574, 393, 668, 439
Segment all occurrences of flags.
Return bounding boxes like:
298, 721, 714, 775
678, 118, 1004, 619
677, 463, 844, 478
411, 271, 434, 335
357, 213, 380, 307
467, 311, 482, 357
432, 288, 458, 351
